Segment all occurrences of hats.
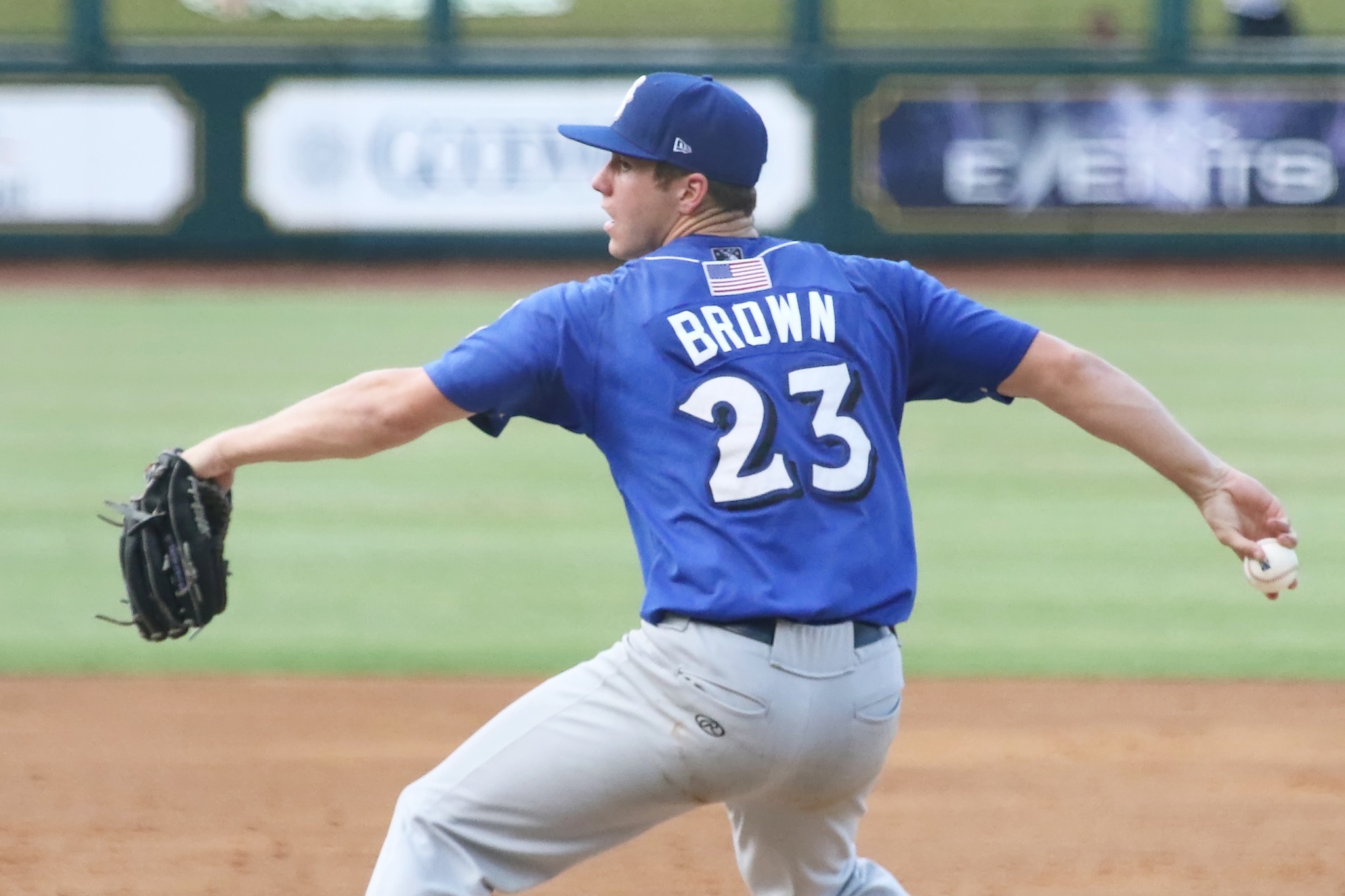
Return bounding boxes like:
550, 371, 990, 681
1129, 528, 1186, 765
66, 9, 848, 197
558, 71, 769, 188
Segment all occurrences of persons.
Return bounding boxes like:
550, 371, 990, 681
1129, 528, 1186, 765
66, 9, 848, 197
94, 72, 1300, 895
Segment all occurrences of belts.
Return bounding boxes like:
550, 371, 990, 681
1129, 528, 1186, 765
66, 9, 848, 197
714, 618, 885, 650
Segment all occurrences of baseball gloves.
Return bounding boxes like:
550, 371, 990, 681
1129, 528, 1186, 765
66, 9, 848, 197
117, 449, 232, 644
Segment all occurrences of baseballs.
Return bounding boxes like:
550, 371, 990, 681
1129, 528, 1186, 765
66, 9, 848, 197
1242, 536, 1300, 592
